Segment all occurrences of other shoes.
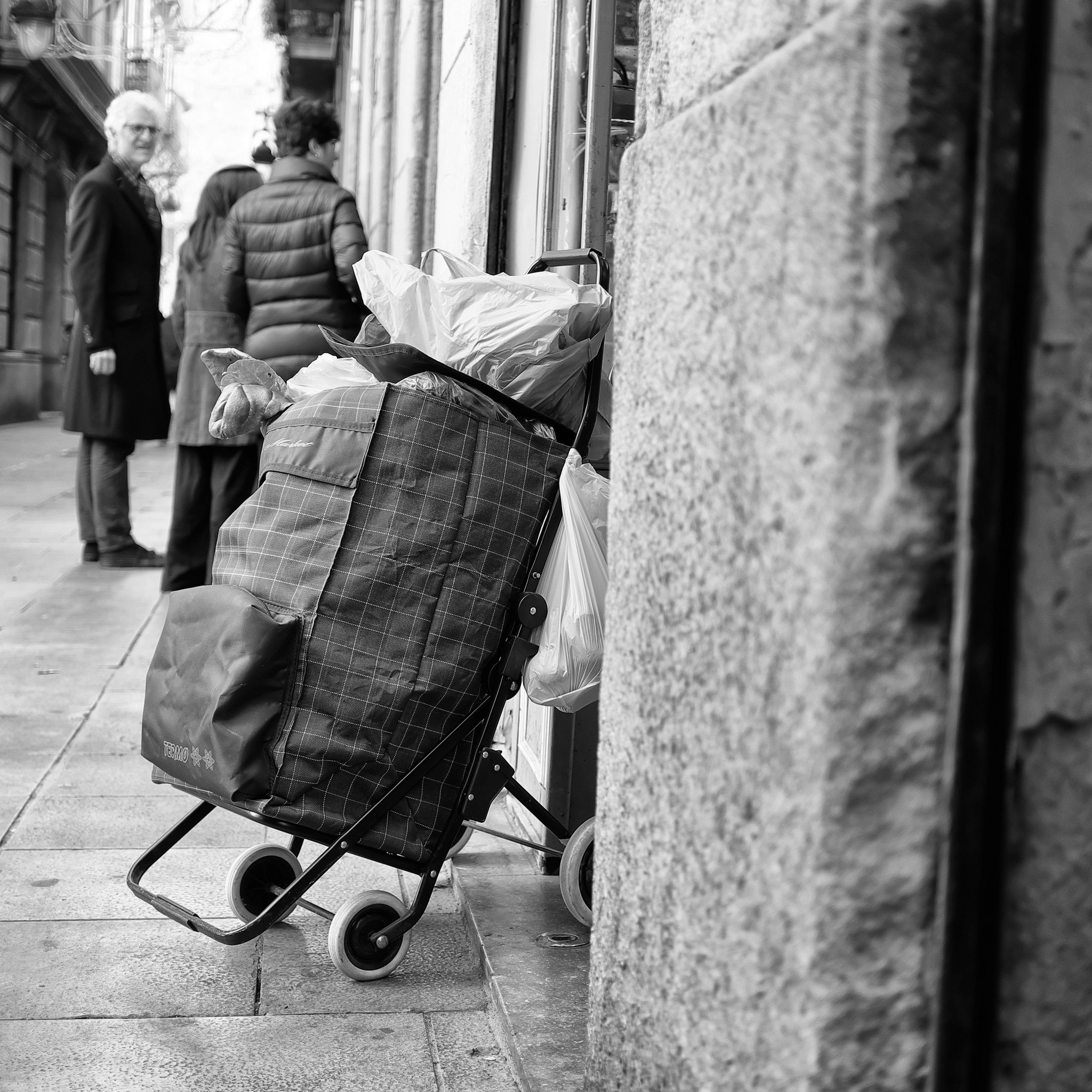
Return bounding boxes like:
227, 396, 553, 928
83, 541, 99, 561
100, 542, 165, 567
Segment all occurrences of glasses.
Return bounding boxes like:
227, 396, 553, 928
124, 123, 161, 138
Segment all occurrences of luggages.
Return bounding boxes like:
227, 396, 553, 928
122, 245, 608, 981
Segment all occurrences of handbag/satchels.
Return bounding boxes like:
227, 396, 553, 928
521, 447, 612, 716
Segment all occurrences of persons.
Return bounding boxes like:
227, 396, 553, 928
162, 96, 371, 593
62, 91, 170, 570
